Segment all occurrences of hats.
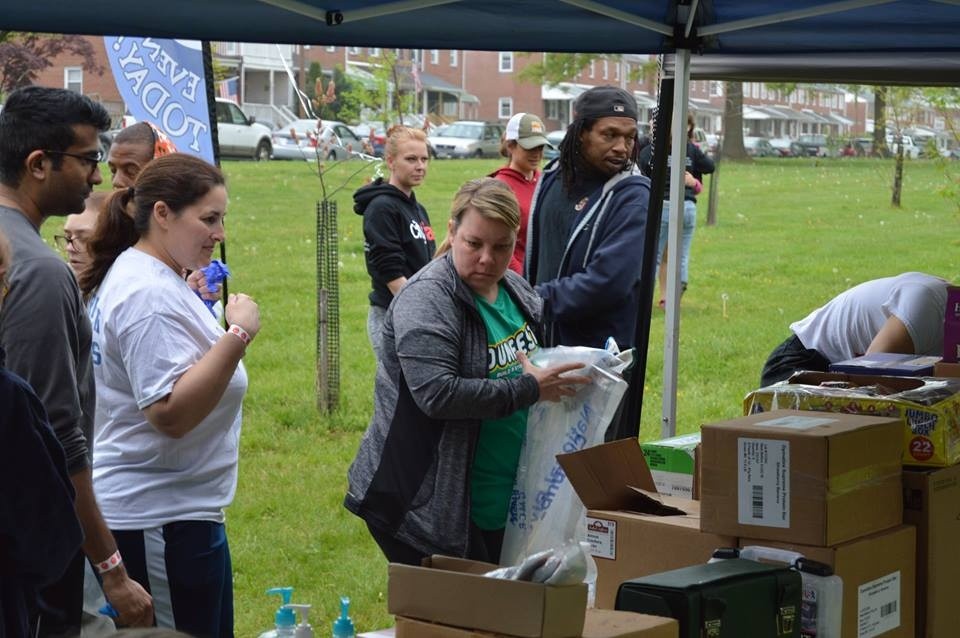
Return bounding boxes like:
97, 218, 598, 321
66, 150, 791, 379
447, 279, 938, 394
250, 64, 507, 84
504, 113, 555, 151
573, 84, 639, 122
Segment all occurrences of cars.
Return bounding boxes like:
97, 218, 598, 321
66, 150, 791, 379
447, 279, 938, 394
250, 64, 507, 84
547, 129, 566, 150
833, 138, 873, 157
273, 118, 365, 161
350, 122, 451, 159
743, 135, 776, 158
691, 128, 720, 155
217, 98, 274, 162
768, 133, 837, 158
887, 134, 960, 159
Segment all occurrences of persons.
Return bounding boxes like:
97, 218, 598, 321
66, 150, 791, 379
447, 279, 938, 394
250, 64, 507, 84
353, 125, 436, 359
760, 272, 959, 387
343, 176, 622, 568
636, 107, 714, 310
487, 112, 554, 275
523, 85, 655, 444
0, 87, 263, 638
844, 144, 856, 155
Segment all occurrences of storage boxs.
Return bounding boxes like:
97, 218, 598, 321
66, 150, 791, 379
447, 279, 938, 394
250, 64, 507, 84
387, 353, 960, 638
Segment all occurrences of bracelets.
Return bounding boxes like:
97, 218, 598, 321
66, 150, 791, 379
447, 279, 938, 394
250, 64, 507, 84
93, 550, 122, 574
227, 324, 252, 346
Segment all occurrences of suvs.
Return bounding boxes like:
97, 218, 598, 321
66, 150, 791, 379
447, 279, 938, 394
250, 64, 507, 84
431, 118, 505, 160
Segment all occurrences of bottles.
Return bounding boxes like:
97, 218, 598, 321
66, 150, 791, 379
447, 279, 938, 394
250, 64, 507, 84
793, 558, 843, 638
705, 548, 740, 566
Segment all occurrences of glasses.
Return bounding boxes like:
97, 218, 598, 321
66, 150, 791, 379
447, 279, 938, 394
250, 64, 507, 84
54, 234, 91, 254
44, 148, 107, 168
